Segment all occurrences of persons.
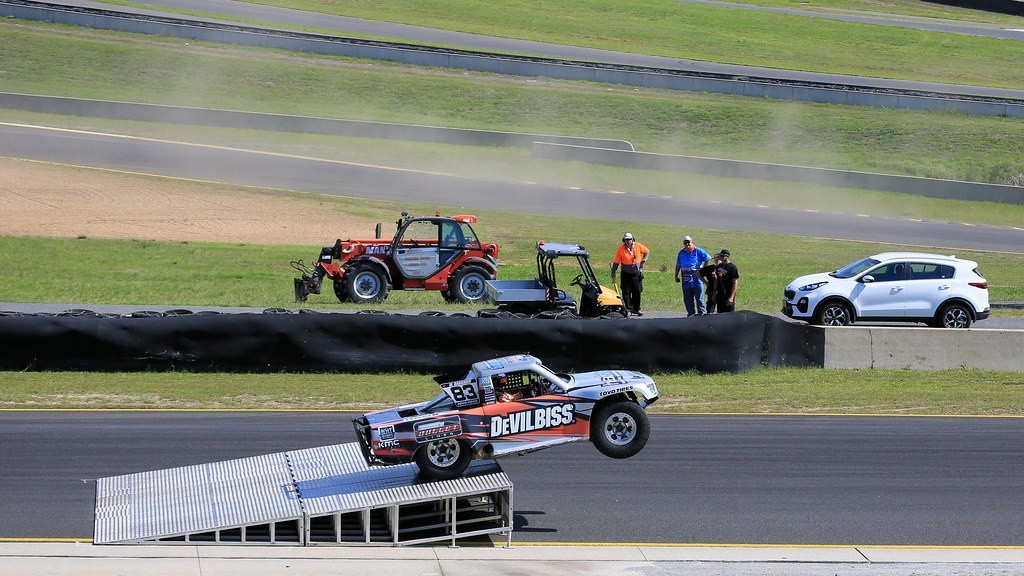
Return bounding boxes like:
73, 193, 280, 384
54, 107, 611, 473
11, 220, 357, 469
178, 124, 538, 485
611, 232, 649, 316
492, 374, 526, 404
697, 254, 722, 314
674, 236, 711, 315
716, 250, 740, 313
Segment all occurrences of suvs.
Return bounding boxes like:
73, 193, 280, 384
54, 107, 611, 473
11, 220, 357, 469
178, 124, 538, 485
781, 252, 991, 329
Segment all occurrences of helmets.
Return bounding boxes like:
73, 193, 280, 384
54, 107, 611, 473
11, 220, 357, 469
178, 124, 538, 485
491, 373, 508, 391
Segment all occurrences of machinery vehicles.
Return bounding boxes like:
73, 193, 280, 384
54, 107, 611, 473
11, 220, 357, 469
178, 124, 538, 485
291, 210, 498, 305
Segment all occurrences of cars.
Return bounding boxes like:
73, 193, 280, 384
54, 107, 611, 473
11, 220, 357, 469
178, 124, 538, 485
350, 351, 658, 480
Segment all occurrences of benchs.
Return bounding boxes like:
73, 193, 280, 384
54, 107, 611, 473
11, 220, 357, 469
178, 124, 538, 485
540, 273, 578, 304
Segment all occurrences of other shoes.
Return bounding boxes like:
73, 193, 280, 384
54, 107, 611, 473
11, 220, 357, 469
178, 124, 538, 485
634, 311, 643, 316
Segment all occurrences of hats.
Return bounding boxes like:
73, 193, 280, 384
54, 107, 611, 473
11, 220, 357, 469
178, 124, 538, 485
712, 253, 722, 259
683, 236, 692, 242
720, 249, 730, 257
624, 233, 633, 240
